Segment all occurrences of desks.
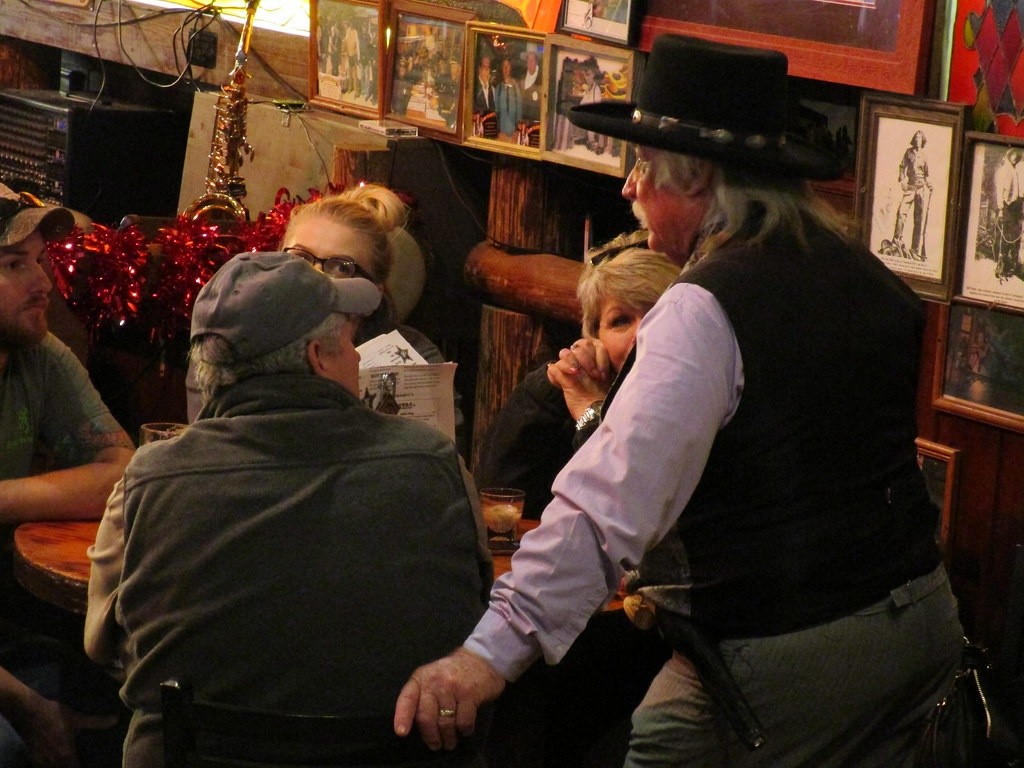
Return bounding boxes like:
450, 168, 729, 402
12, 508, 647, 634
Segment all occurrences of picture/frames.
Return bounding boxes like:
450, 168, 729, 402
915, 439, 964, 551
929, 302, 1024, 432
947, 130, 1024, 313
460, 20, 545, 161
540, 32, 633, 180
941, 0, 1023, 147
854, 93, 970, 303
383, 0, 477, 146
310, 0, 388, 121
633, 0, 936, 97
560, 0, 636, 47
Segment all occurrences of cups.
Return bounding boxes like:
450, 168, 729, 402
140, 423, 188, 446
481, 488, 525, 548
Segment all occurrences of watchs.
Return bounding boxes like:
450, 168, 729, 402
575, 400, 604, 429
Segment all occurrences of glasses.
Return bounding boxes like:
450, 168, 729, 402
0, 192, 47, 223
283, 247, 374, 285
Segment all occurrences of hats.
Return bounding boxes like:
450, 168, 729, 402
0, 182, 74, 248
568, 33, 837, 180
190, 252, 382, 373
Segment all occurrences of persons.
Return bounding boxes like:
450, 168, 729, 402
394, 33, 964, 768
580, 68, 603, 150
476, 251, 680, 517
471, 53, 542, 146
994, 148, 1021, 280
396, 46, 456, 125
316, 13, 377, 102
283, 183, 466, 459
0, 181, 137, 768
893, 131, 933, 261
84, 250, 493, 768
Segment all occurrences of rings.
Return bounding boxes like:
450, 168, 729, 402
439, 709, 456, 717
